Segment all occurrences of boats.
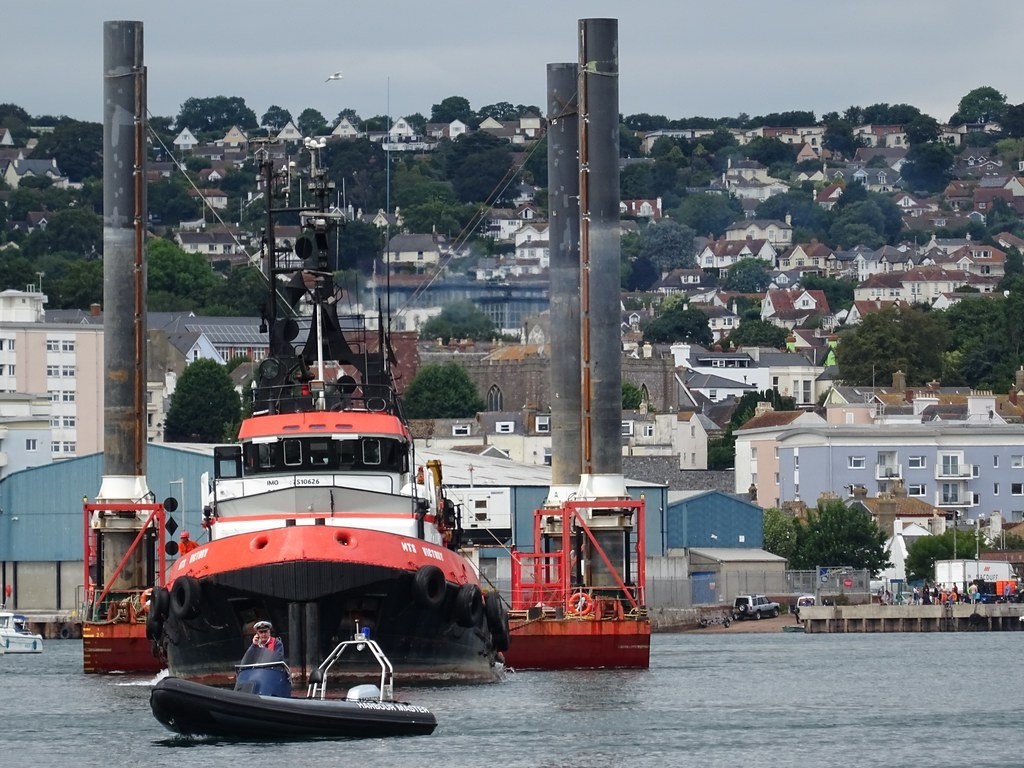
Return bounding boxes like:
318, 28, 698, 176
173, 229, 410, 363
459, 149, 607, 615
0, 604, 43, 654
148, 619, 438, 742
142, 161, 510, 687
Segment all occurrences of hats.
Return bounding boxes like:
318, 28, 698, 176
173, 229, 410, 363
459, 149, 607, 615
253, 621, 272, 631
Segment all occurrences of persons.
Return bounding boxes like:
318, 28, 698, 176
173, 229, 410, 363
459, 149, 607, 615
880, 580, 960, 604
240, 621, 285, 673
966, 582, 978, 604
1002, 581, 1012, 605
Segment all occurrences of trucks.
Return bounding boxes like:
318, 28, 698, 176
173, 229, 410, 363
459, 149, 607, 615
934, 559, 1021, 603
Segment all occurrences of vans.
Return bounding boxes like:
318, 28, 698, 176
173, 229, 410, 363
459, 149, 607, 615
794, 596, 815, 622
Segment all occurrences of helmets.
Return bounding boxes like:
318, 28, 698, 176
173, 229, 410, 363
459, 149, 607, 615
180, 532, 189, 539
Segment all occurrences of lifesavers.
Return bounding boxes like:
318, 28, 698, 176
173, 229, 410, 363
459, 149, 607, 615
141, 588, 154, 613
568, 592, 593, 616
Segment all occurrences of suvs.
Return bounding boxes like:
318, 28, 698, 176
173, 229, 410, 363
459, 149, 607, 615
731, 593, 780, 620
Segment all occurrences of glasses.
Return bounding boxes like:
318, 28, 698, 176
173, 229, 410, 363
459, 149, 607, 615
257, 630, 269, 633
